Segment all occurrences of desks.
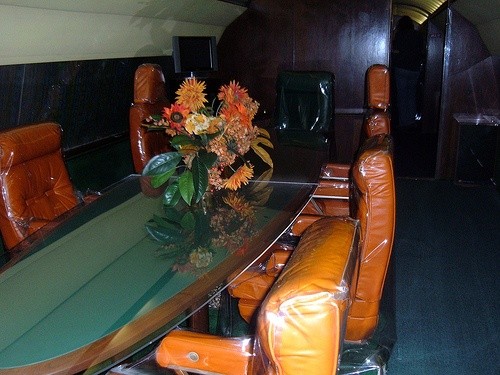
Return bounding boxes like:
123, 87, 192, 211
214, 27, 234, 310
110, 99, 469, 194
450, 123, 500, 202
0, 146, 329, 375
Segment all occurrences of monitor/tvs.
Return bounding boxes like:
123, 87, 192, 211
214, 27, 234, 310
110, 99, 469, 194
172, 35, 218, 73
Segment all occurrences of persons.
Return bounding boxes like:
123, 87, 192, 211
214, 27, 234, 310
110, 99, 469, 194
390, 15, 425, 131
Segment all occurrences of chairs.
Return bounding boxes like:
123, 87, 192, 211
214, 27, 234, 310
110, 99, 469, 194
0, 65, 399, 375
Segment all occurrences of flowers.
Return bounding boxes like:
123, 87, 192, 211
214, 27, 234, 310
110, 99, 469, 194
143, 170, 274, 281
141, 71, 273, 215
